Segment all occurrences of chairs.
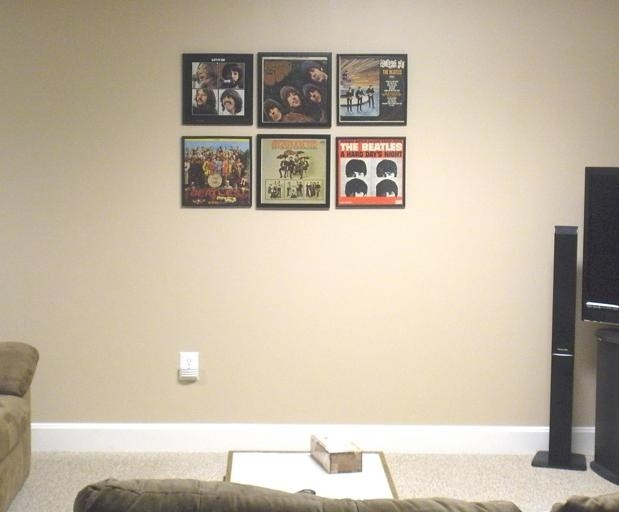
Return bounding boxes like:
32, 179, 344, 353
0, 341, 39, 511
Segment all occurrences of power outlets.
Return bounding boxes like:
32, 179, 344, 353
179, 352, 199, 381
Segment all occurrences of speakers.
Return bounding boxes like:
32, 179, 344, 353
531, 225, 586, 471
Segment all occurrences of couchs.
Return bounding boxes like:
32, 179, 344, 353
74, 478, 619, 512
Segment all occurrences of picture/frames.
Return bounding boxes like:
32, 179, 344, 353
335, 136, 406, 209
181, 136, 253, 207
336, 53, 408, 126
257, 135, 330, 208
181, 53, 254, 124
257, 51, 332, 129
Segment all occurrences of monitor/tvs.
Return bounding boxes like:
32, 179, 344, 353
581, 167, 619, 326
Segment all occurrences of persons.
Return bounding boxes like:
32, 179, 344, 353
183, 61, 398, 205
